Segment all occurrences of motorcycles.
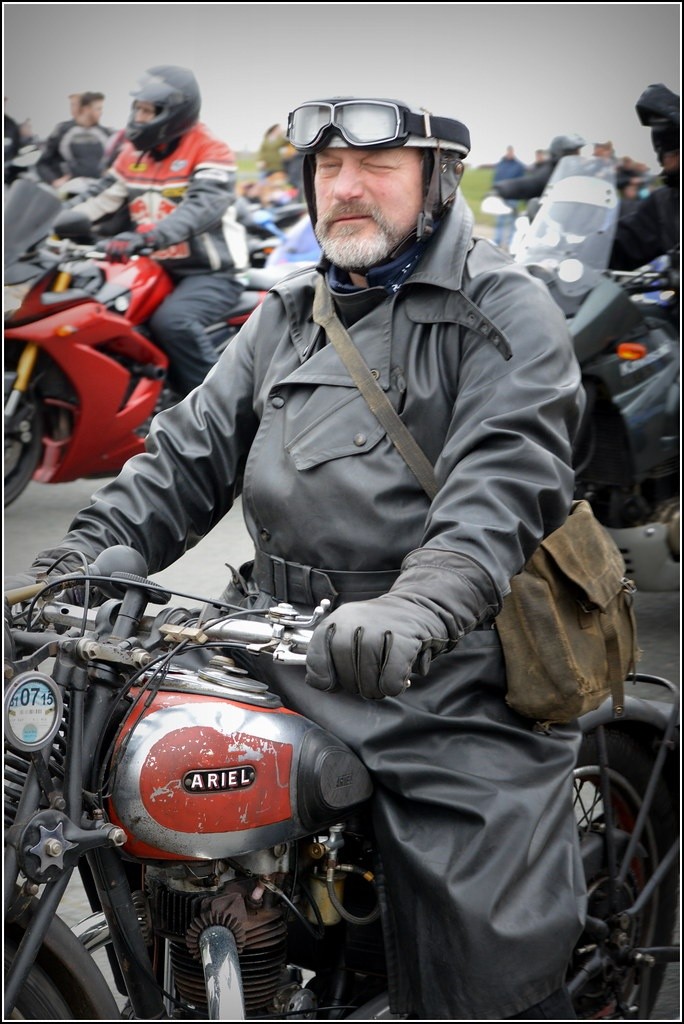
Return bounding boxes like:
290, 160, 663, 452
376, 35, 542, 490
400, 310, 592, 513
0, 546, 681, 1018
5, 173, 65, 313
516, 157, 678, 557
4, 213, 291, 504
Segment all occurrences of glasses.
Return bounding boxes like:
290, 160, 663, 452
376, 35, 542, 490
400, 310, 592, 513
287, 101, 471, 153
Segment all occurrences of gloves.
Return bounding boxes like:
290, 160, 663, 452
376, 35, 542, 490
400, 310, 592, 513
306, 547, 504, 701
104, 228, 169, 266
5, 548, 105, 635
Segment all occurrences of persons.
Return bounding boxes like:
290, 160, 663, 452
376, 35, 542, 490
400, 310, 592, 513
0, 61, 679, 1020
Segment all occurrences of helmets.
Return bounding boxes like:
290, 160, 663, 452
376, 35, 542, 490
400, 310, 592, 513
127, 64, 200, 151
551, 136, 586, 159
283, 42, 474, 161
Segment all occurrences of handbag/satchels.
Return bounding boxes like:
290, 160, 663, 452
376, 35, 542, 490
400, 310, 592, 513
494, 499, 640, 721
